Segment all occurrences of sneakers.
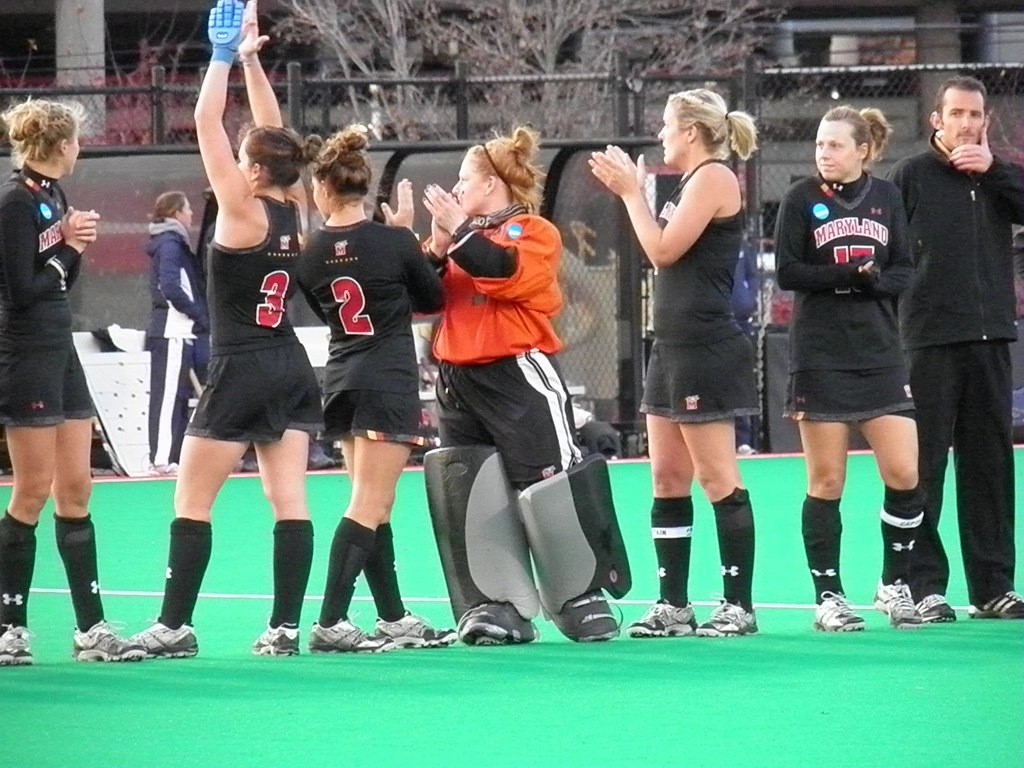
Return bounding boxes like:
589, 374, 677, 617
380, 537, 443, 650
626, 599, 698, 637
813, 590, 865, 631
873, 578, 923, 629
251, 619, 300, 655
915, 594, 956, 624
696, 598, 758, 636
0, 623, 36, 665
73, 619, 147, 662
967, 590, 1024, 619
374, 613, 459, 650
129, 621, 199, 658
308, 618, 395, 653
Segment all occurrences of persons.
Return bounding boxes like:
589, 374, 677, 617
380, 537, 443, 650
127, 0, 326, 660
296, 126, 456, 654
887, 77, 1024, 623
0, 99, 148, 664
589, 88, 762, 637
774, 106, 915, 632
423, 130, 633, 643
145, 191, 207, 478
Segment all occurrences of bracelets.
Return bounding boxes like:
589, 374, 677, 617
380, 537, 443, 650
48, 255, 69, 287
